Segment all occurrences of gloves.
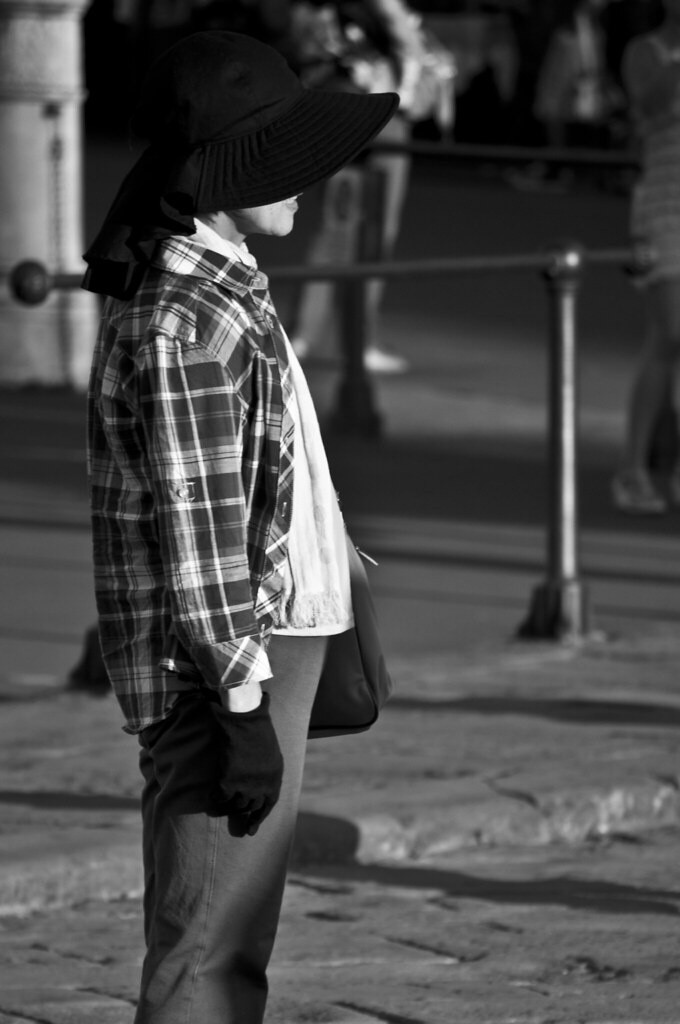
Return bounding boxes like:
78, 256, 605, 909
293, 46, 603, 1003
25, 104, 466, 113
205, 688, 284, 837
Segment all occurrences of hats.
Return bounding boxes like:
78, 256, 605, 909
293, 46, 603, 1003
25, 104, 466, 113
81, 33, 401, 303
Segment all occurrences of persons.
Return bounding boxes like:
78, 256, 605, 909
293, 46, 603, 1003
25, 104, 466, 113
85, 32, 400, 1022
272, 0, 456, 440
534, 1, 628, 188
613, 0, 680, 512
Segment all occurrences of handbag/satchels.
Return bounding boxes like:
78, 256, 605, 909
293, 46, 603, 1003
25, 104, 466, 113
307, 534, 391, 739
